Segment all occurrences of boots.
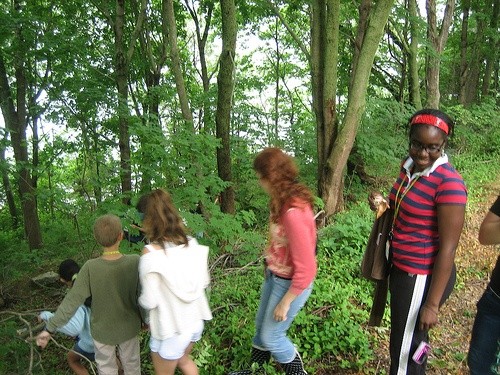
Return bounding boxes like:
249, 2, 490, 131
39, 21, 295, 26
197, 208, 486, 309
280, 349, 308, 375
228, 344, 271, 375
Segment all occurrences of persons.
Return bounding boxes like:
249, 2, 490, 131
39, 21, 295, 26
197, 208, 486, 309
248, 147, 318, 375
367, 109, 468, 375
36, 214, 147, 375
39, 259, 95, 375
135, 189, 214, 375
467, 194, 500, 375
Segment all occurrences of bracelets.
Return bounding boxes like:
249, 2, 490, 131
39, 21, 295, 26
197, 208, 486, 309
44, 325, 53, 334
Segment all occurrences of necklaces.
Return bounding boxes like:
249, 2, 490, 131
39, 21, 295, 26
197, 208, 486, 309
392, 166, 430, 225
103, 251, 121, 254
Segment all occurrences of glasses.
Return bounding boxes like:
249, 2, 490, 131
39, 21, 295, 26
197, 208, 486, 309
409, 134, 446, 153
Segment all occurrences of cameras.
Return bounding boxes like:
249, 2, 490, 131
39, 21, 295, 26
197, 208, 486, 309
412, 341, 431, 365
